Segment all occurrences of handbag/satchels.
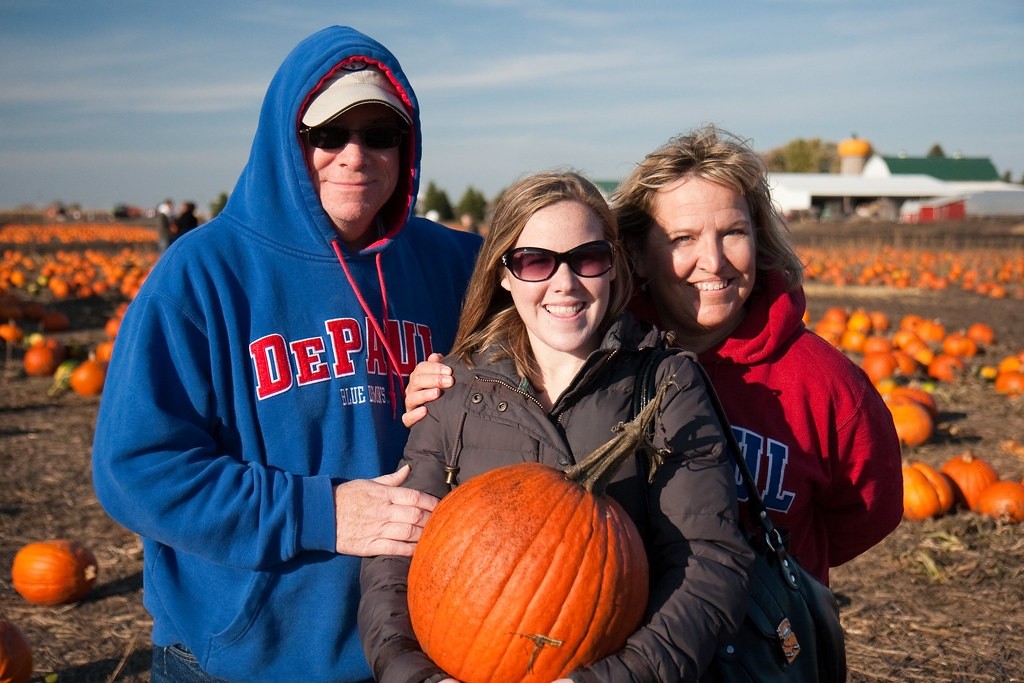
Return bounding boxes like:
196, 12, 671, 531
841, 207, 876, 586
631, 347, 847, 683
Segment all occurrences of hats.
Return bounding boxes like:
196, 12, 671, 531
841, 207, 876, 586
301, 63, 415, 127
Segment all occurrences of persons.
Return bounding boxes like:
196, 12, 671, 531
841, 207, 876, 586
359, 172, 768, 683
90, 25, 484, 683
402, 128, 906, 590
156, 192, 228, 251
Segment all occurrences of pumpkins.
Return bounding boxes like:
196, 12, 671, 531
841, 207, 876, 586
1, 620, 34, 683
407, 462, 649, 683
785, 239, 1024, 523
12, 538, 99, 605
1, 223, 162, 398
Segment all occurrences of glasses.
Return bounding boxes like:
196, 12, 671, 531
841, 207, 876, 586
499, 240, 616, 283
299, 117, 409, 150
